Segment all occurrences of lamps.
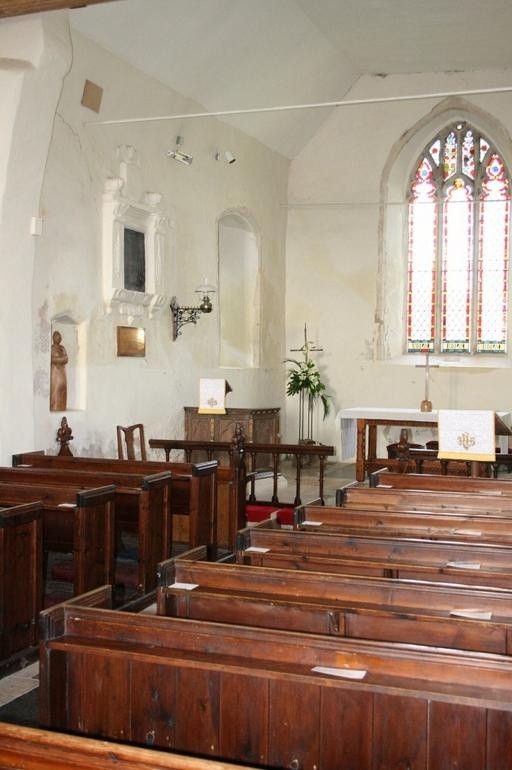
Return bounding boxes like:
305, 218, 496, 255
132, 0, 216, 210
170, 277, 216, 341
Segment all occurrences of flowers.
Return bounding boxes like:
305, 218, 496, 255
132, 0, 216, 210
282, 341, 332, 422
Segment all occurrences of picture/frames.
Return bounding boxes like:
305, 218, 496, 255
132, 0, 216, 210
117, 326, 145, 357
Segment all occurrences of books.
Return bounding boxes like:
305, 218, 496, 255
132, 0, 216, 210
450, 608, 492, 621
445, 560, 481, 572
310, 664, 367, 682
453, 528, 482, 538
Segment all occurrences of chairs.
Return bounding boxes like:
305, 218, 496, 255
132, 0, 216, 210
117, 424, 146, 461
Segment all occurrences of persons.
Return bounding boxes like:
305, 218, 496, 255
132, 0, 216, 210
51, 331, 68, 407
